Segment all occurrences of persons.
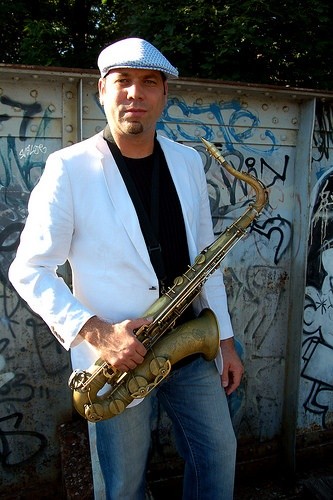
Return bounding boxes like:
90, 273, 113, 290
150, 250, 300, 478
8, 37, 244, 500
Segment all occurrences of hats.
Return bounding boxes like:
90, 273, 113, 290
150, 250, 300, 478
97, 38, 179, 79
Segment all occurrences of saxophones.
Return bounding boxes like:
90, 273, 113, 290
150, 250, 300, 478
66, 136, 272, 422
